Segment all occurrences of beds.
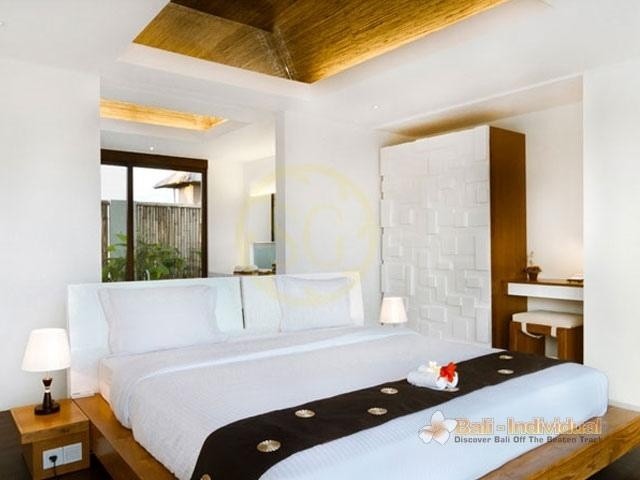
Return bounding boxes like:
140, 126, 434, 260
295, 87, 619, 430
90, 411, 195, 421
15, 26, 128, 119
66, 272, 639, 479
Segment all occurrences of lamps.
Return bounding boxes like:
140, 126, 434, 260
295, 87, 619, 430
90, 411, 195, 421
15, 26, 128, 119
379, 298, 408, 323
20, 328, 71, 416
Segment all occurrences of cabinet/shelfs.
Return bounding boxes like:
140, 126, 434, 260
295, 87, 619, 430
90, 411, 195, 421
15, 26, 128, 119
378, 126, 528, 348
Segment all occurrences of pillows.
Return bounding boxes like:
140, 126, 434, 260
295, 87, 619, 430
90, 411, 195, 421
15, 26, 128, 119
276, 277, 352, 330
99, 285, 227, 354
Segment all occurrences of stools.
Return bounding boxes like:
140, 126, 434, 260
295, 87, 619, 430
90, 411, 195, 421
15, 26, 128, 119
509, 309, 582, 361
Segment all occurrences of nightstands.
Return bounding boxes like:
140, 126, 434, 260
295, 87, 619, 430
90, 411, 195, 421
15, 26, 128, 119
9, 401, 89, 480
